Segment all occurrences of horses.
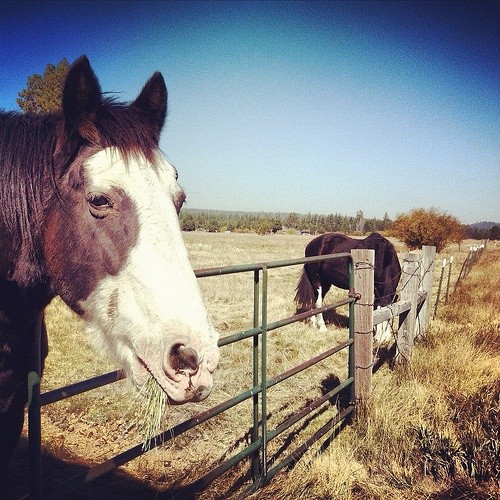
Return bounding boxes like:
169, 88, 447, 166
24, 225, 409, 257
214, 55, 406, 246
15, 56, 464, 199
0, 53, 221, 500
293, 233, 402, 346
